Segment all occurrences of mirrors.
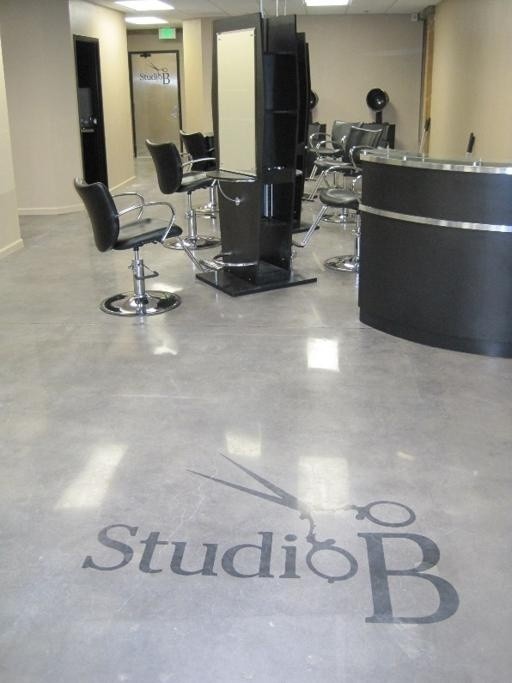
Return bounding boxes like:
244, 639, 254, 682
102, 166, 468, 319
215, 27, 257, 177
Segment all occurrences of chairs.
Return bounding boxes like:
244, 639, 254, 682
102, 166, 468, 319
179, 129, 221, 213
74, 176, 183, 317
289, 120, 394, 273
205, 135, 214, 148
145, 139, 223, 250
